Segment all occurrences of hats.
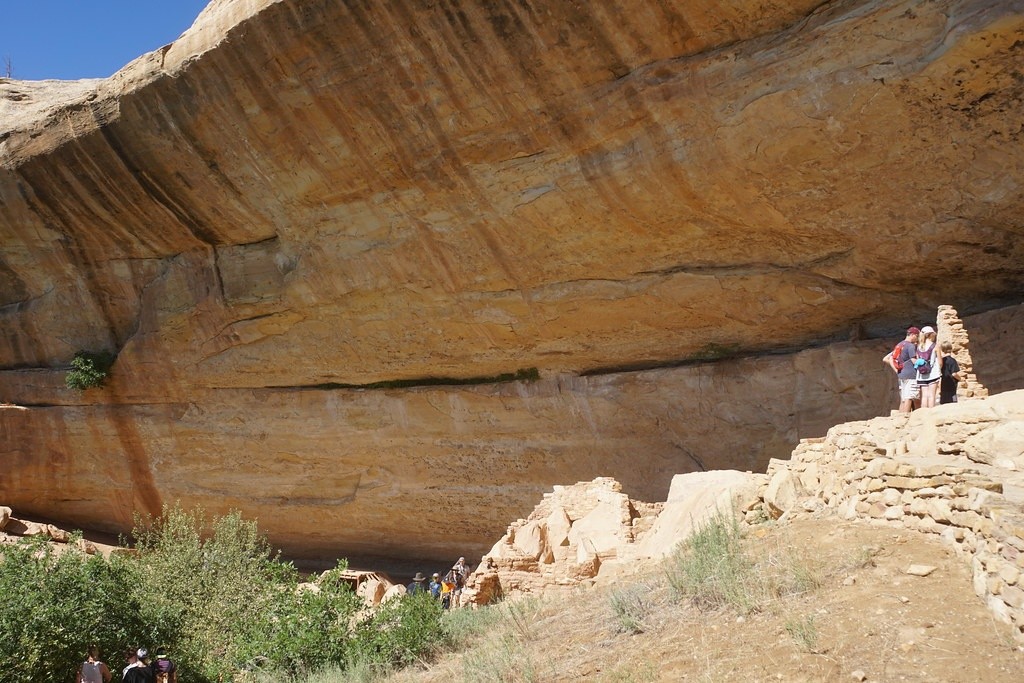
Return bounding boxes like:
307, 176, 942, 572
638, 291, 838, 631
450, 566, 460, 571
444, 577, 449, 582
413, 573, 425, 581
922, 326, 936, 335
908, 327, 921, 334
433, 573, 440, 578
137, 648, 148, 658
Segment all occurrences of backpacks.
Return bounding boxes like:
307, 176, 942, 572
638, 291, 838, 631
915, 343, 936, 374
892, 341, 911, 370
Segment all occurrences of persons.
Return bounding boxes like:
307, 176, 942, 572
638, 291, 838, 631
940, 341, 961, 404
916, 326, 943, 408
406, 557, 471, 609
882, 327, 920, 412
77, 645, 177, 683
